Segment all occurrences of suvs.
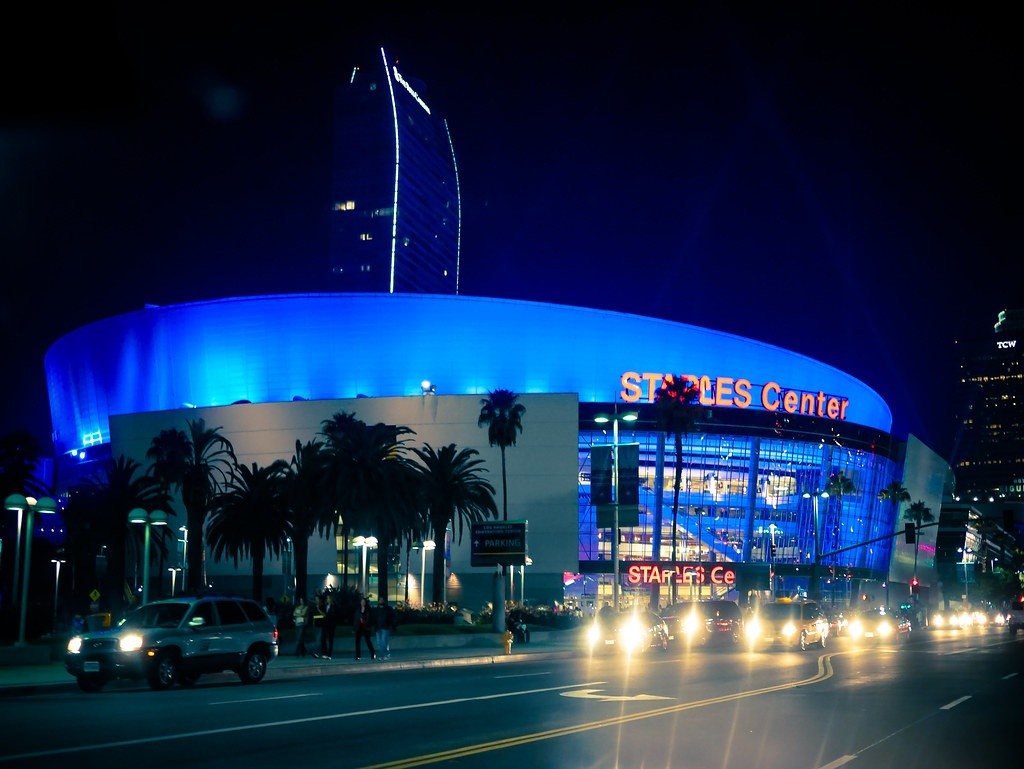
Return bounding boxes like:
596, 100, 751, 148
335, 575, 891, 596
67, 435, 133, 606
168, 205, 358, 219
63, 593, 280, 693
1008, 600, 1024, 635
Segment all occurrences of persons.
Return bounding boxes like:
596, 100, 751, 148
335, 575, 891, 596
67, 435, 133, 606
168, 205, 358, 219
293, 594, 340, 660
508, 610, 530, 643
352, 596, 395, 661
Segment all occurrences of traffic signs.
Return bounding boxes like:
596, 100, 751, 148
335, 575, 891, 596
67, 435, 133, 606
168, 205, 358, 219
470, 522, 527, 567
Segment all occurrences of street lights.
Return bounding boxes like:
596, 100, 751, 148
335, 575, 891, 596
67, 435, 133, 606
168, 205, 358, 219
127, 508, 169, 606
592, 411, 637, 613
957, 548, 973, 594
50, 559, 66, 589
168, 567, 182, 597
178, 526, 190, 593
412, 539, 437, 607
803, 487, 830, 602
4, 494, 57, 650
352, 535, 379, 600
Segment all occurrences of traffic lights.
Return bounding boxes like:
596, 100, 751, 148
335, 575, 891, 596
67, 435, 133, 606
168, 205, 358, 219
912, 579, 919, 593
771, 544, 777, 557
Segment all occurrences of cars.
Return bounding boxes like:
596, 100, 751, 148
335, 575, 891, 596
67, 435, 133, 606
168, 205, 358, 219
589, 609, 671, 658
850, 608, 913, 646
931, 606, 1007, 631
748, 598, 828, 652
660, 600, 743, 649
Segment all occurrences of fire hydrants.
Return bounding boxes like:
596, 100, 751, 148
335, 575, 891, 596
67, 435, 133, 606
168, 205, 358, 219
503, 629, 514, 655
